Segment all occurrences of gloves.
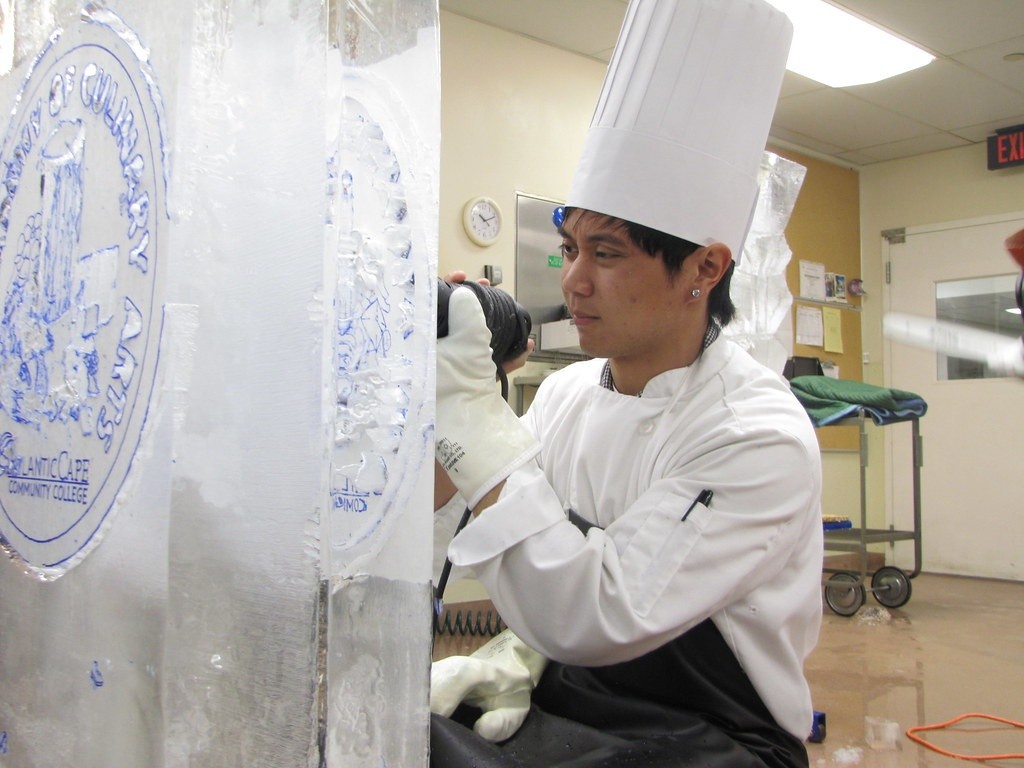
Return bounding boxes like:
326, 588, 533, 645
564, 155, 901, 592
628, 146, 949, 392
433, 287, 546, 509
430, 626, 551, 743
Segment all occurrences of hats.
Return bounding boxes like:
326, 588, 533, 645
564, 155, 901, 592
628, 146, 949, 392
561, 1, 794, 264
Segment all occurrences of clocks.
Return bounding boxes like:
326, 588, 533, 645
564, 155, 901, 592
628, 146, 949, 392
462, 197, 503, 247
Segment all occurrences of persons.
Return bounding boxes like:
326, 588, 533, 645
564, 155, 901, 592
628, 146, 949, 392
431, 1, 823, 767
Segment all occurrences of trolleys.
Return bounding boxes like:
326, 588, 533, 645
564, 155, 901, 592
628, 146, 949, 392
796, 372, 926, 616
